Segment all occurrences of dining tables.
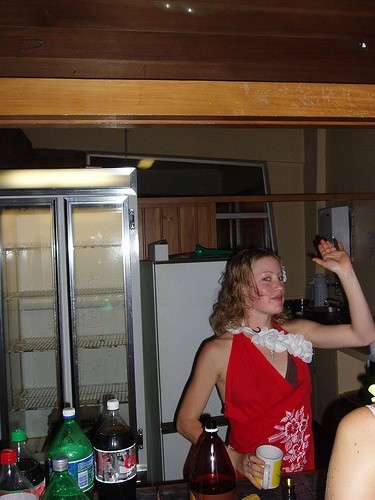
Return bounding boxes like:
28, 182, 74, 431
89, 471, 317, 500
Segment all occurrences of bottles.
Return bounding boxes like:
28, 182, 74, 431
10, 427, 46, 500
46, 407, 94, 500
94, 399, 137, 500
39, 456, 89, 500
189, 420, 237, 500
0, 449, 39, 500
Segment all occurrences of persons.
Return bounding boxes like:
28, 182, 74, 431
176, 239, 375, 490
325, 384, 375, 500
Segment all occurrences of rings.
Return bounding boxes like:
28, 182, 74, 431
249, 462, 253, 468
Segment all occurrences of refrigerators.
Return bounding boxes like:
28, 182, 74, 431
140, 259, 228, 481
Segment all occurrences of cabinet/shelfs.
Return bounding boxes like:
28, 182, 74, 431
311, 345, 372, 433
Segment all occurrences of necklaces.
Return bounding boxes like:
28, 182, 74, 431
248, 334, 275, 361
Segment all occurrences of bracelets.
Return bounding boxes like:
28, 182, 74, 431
227, 445, 234, 452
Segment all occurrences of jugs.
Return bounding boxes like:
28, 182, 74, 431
309, 273, 330, 307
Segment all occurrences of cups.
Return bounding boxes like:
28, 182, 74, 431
254, 445, 283, 489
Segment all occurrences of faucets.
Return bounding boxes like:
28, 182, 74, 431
324, 297, 343, 311
